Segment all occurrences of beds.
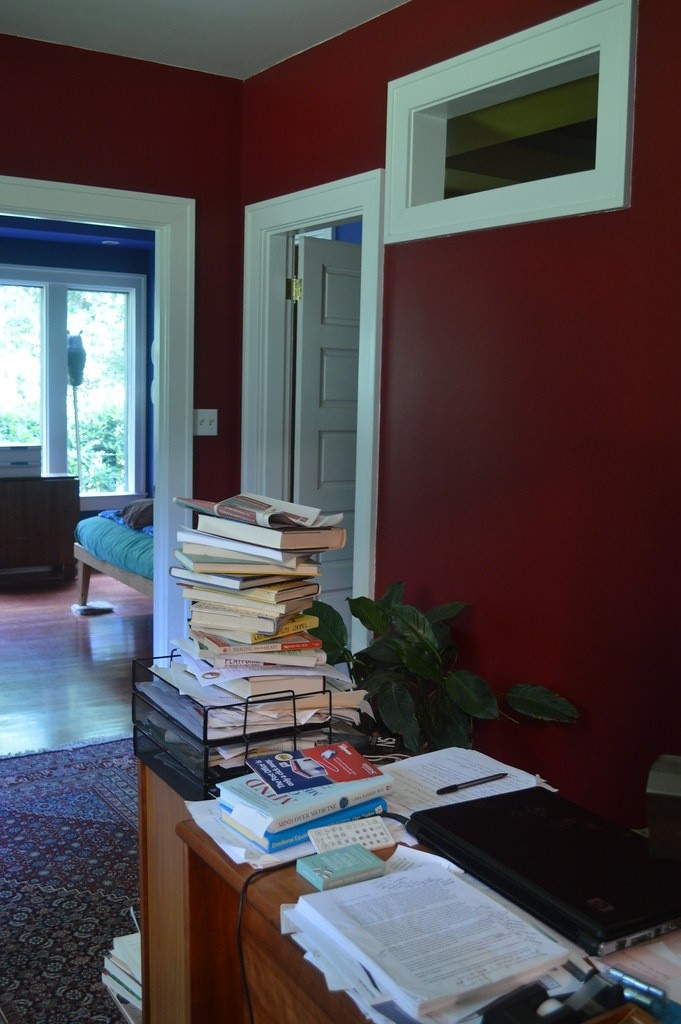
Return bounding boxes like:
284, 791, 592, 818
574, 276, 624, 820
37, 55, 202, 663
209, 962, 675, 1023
69, 507, 154, 604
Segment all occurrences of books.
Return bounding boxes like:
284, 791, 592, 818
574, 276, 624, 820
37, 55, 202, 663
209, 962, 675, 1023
168, 515, 681, 1016
102, 905, 142, 1024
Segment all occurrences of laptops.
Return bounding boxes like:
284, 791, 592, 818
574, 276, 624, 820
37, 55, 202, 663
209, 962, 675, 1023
406, 786, 681, 958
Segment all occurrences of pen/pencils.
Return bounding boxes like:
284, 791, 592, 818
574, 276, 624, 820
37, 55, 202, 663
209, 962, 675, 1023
437, 772, 508, 794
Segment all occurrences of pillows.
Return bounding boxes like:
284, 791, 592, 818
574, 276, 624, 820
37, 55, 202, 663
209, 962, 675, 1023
113, 499, 154, 531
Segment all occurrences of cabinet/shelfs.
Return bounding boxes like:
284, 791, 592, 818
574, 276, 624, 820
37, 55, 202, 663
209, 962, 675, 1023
137, 756, 377, 1024
0, 476, 81, 580
